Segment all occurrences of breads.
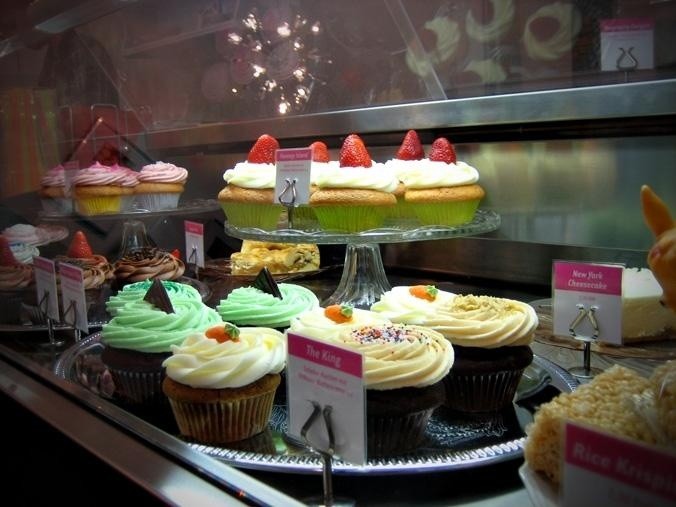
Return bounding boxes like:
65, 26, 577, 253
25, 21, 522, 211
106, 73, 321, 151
229, 239, 320, 276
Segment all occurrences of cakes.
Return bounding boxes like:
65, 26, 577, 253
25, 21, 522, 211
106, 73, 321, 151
114, 248, 185, 296
214, 268, 319, 405
9, 244, 40, 266
100, 277, 225, 410
370, 285, 457, 323
464, 0, 516, 45
52, 231, 116, 323
320, 324, 456, 458
456, 59, 507, 89
0, 237, 34, 325
164, 320, 288, 444
105, 281, 202, 318
386, 129, 425, 226
309, 134, 396, 234
621, 267, 676, 341
419, 294, 539, 425
40, 165, 72, 216
110, 163, 138, 211
405, 138, 484, 227
522, 2, 584, 63
134, 161, 189, 211
284, 304, 394, 334
71, 161, 127, 217
291, 141, 340, 229
1, 224, 51, 259
404, 16, 462, 76
218, 135, 281, 230
37, 223, 69, 257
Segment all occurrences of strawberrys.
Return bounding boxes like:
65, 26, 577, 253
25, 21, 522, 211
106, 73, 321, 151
0, 237, 16, 267
